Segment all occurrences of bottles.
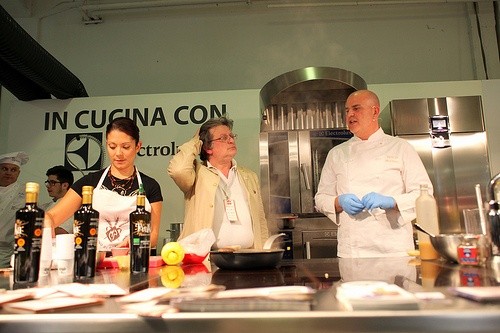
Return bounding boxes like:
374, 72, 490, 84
14, 182, 45, 284
456, 235, 481, 267
415, 183, 439, 261
74, 186, 100, 281
130, 195, 151, 274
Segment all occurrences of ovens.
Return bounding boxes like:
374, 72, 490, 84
257, 128, 354, 258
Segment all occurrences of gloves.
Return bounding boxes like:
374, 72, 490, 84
361, 192, 394, 211
337, 194, 364, 216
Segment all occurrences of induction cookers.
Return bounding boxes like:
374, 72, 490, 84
209, 258, 499, 312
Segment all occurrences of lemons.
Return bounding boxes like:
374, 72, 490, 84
161, 241, 184, 265
161, 265, 185, 288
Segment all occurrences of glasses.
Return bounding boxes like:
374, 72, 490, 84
208, 134, 238, 142
45, 180, 66, 186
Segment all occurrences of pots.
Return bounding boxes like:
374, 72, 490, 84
209, 232, 287, 272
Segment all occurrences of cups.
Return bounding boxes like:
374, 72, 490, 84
96, 250, 105, 264
42, 227, 74, 277
111, 248, 129, 257
463, 207, 492, 260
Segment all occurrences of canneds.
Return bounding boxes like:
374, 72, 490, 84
457, 235, 483, 265
459, 265, 483, 287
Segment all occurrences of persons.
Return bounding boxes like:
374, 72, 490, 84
315, 89, 434, 259
168, 113, 274, 250
338, 258, 417, 285
43, 117, 164, 248
0, 151, 74, 268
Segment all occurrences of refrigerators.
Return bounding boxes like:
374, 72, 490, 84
389, 94, 491, 235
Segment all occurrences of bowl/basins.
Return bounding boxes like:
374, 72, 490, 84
429, 233, 490, 263
160, 241, 209, 266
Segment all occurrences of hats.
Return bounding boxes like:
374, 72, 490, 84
0, 151, 28, 169
